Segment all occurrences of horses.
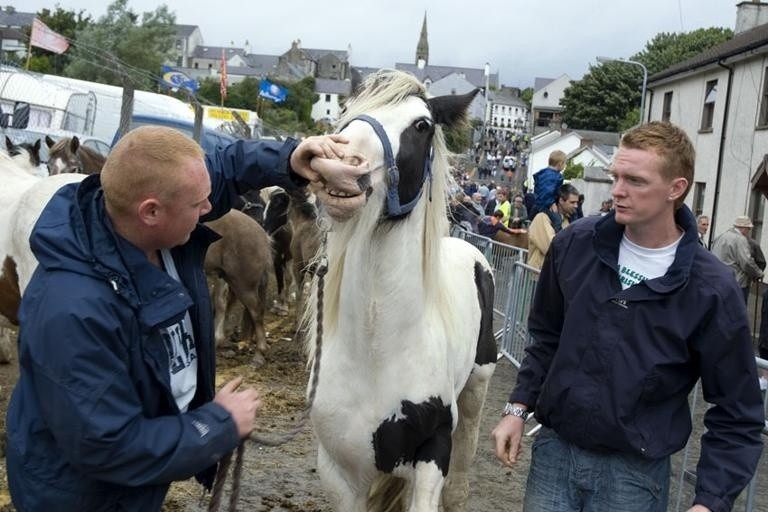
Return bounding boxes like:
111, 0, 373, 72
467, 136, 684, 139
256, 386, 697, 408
44, 134, 292, 370
292, 66, 498, 512
0, 148, 90, 364
288, 188, 325, 337
496, 230, 529, 252
3, 134, 43, 179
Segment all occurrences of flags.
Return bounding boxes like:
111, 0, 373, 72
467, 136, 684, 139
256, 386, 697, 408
30, 19, 70, 54
260, 77, 289, 104
220, 55, 226, 97
158, 66, 199, 97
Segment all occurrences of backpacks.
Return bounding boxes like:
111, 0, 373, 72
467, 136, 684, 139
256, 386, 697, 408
748, 238, 767, 272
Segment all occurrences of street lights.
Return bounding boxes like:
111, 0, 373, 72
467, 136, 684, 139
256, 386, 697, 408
595, 56, 648, 128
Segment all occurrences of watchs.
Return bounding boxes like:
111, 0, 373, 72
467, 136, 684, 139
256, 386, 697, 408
500, 402, 529, 419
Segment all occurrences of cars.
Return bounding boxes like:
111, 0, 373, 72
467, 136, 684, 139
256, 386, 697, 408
1, 122, 112, 177
503, 156, 517, 171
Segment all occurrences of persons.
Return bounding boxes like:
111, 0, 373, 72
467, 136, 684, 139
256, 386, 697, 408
4, 125, 350, 512
693, 213, 709, 250
711, 216, 765, 293
447, 127, 612, 330
742, 235, 766, 305
494, 120, 766, 511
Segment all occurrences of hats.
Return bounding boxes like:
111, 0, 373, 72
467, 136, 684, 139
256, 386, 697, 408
732, 215, 756, 228
